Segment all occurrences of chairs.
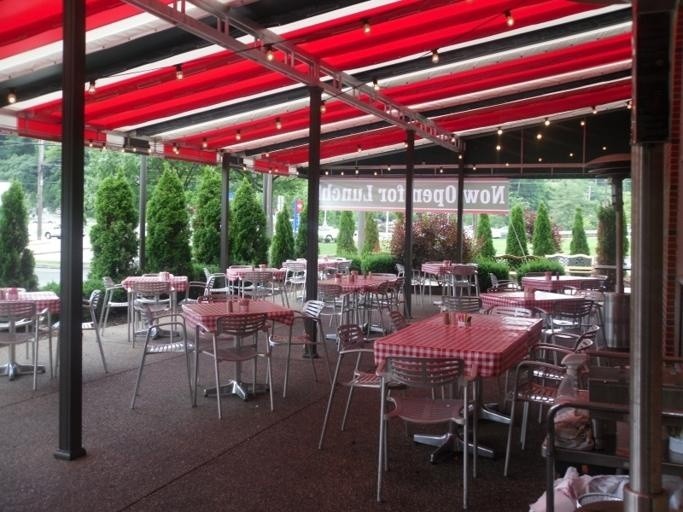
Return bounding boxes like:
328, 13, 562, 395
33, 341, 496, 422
377, 356, 478, 511
0, 285, 108, 391
95, 259, 607, 347
380, 307, 600, 455
319, 323, 406, 474
130, 294, 329, 420
501, 338, 594, 478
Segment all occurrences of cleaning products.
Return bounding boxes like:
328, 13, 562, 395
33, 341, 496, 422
555, 353, 591, 417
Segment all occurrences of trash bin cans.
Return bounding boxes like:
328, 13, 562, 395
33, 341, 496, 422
604, 292, 630, 351
576, 493, 624, 511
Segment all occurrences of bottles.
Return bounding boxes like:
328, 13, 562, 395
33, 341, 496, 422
351, 275, 354, 282
226, 296, 232, 312
251, 265, 254, 271
0, 287, 18, 303
449, 311, 457, 327
556, 272, 559, 279
363, 271, 371, 279
159, 270, 169, 281
441, 308, 449, 325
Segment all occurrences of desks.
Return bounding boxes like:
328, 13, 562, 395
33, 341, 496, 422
373, 311, 543, 463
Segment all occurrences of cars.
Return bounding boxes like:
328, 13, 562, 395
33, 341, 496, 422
361, 220, 525, 239
317, 225, 338, 242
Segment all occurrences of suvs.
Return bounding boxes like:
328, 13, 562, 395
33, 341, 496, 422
25, 205, 86, 240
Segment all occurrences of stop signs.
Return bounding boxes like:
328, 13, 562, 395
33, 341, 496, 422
295, 199, 305, 211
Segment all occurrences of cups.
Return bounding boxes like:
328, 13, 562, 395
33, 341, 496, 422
238, 304, 248, 314
544, 271, 551, 280
333, 273, 342, 282
258, 264, 265, 272
442, 260, 448, 269
350, 270, 358, 280
